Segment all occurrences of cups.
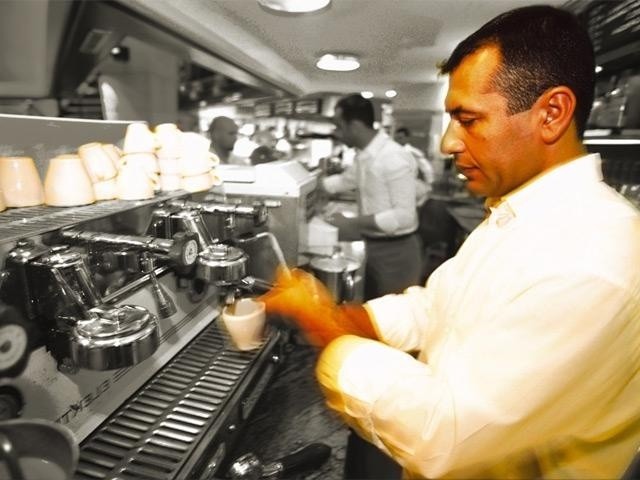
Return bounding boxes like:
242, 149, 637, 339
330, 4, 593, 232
221, 299, 268, 352
1, 122, 224, 212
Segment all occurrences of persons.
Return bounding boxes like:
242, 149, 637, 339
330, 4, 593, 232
249, 130, 286, 168
253, 2, 640, 480
306, 91, 424, 298
204, 117, 248, 168
392, 126, 437, 187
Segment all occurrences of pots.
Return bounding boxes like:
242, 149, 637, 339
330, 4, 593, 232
310, 254, 361, 303
